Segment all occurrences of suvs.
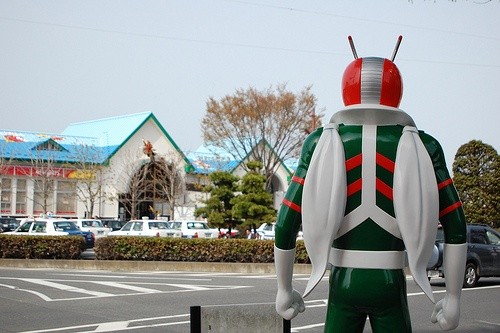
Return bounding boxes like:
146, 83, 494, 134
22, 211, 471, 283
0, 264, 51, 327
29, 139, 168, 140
412, 223, 500, 288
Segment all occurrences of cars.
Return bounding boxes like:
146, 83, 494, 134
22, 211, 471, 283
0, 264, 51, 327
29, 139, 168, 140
99, 219, 132, 232
0, 218, 96, 254
249, 221, 306, 242
169, 219, 219, 240
67, 219, 112, 242
218, 224, 241, 239
107, 218, 184, 242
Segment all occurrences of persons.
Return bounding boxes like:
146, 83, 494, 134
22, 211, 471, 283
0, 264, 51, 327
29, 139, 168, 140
246, 230, 254, 240
254, 233, 261, 241
274, 35, 468, 333
